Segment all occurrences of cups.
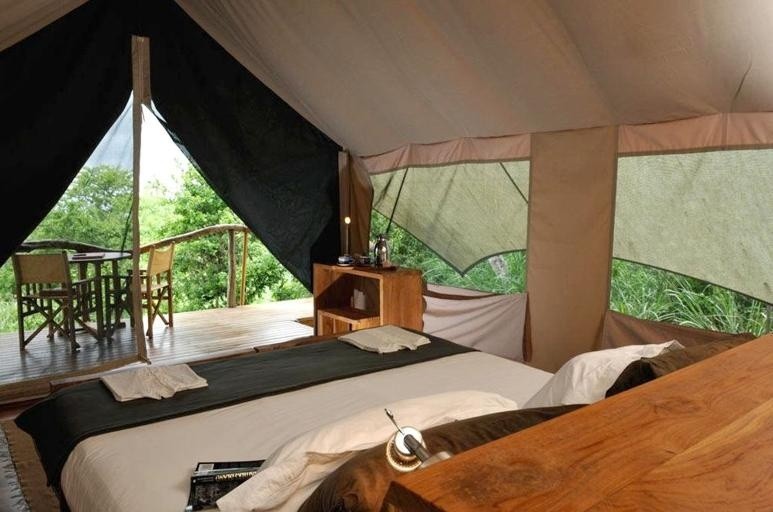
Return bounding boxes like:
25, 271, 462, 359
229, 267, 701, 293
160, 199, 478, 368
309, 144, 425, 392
339, 257, 350, 264
394, 426, 423, 461
359, 258, 371, 265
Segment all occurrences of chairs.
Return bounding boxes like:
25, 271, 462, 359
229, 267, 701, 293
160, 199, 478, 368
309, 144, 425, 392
10, 251, 98, 358
103, 241, 174, 345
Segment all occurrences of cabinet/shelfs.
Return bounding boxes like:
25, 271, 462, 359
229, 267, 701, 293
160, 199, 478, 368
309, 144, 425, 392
313, 262, 424, 336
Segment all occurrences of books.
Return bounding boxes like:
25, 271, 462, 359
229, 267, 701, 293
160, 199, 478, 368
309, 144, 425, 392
182, 457, 268, 511
71, 252, 106, 258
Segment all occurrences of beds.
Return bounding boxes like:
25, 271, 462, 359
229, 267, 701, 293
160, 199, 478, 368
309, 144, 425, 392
13, 325, 773, 512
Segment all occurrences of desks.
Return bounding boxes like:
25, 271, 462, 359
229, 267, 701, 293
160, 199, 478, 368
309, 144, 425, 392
67, 252, 131, 343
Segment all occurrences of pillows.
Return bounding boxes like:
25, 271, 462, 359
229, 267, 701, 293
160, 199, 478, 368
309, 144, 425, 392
520, 338, 688, 401
217, 388, 519, 512
299, 403, 591, 512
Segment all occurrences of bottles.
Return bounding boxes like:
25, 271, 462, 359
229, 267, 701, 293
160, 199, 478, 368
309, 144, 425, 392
377, 235, 387, 269
356, 292, 366, 310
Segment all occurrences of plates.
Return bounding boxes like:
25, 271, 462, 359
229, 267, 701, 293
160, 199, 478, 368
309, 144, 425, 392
338, 264, 352, 267
